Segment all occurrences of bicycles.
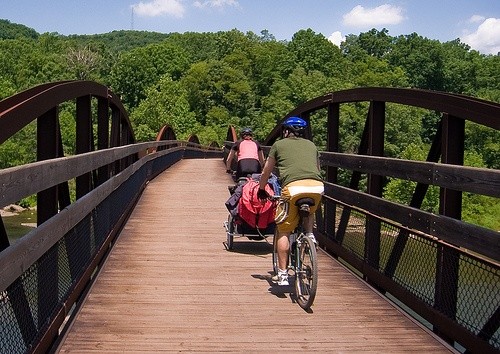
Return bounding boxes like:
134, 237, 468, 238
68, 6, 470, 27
226, 163, 261, 182
256, 190, 318, 310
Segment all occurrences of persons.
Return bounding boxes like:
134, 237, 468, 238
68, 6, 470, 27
257, 117, 325, 286
226, 127, 266, 183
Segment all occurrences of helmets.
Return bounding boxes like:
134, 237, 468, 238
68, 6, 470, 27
282, 117, 308, 132
240, 127, 253, 135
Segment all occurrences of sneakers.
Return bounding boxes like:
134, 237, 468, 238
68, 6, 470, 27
306, 236, 319, 255
271, 271, 290, 287
